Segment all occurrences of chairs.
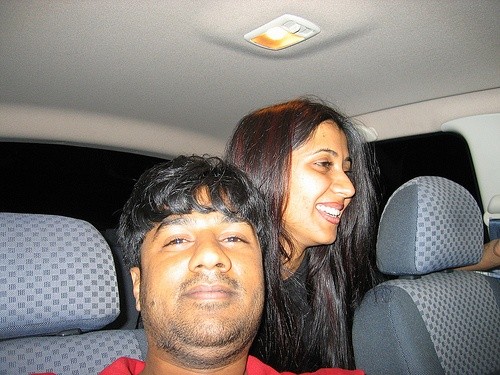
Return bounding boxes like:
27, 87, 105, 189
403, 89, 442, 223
0, 212, 149, 375
351, 176, 500, 375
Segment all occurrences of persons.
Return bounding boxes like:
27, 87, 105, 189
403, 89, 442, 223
26, 154, 364, 374
223, 93, 499, 374
